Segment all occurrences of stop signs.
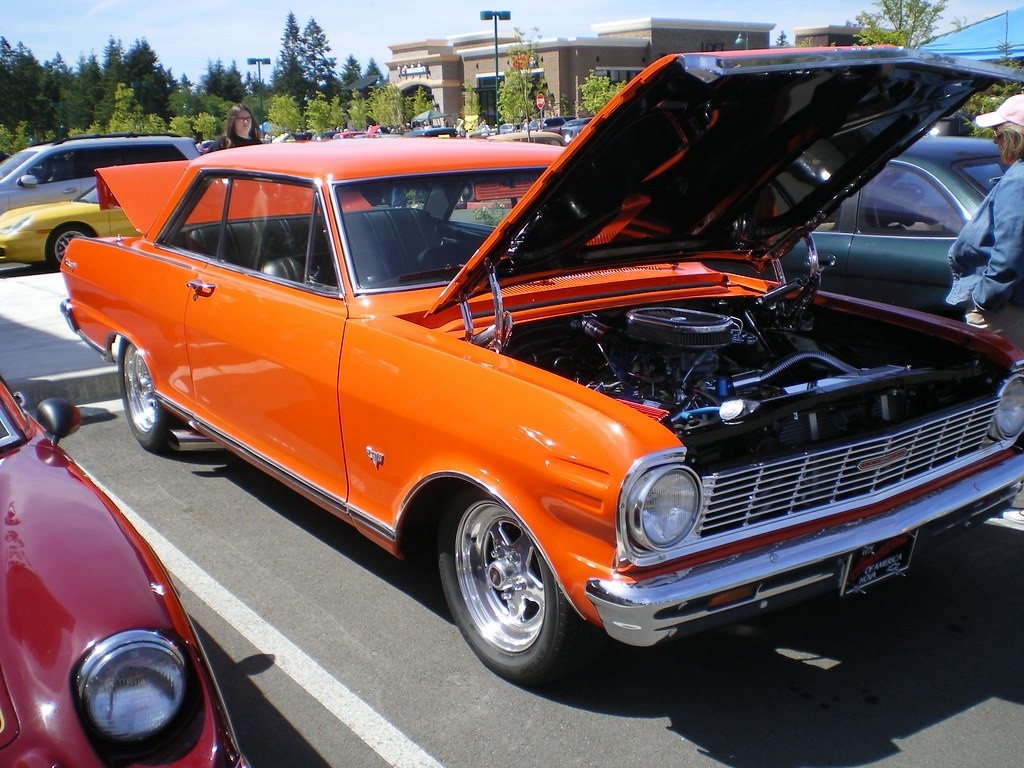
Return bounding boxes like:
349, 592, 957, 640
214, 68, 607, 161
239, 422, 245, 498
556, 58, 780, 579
536, 91, 545, 110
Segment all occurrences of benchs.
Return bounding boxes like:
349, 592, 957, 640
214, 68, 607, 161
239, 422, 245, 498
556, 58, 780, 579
187, 208, 441, 279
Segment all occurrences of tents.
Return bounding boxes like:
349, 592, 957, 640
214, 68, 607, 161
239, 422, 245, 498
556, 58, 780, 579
410, 109, 452, 129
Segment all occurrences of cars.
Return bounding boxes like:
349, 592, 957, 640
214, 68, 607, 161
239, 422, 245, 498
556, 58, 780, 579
709, 135, 1011, 324
61, 43, 1024, 691
319, 115, 594, 147
0, 181, 143, 271
1, 376, 251, 768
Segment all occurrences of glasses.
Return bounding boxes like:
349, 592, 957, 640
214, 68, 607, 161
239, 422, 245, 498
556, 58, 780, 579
994, 129, 1003, 138
235, 115, 252, 123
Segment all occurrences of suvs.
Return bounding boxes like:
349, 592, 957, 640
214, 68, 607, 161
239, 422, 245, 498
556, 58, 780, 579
0, 129, 204, 215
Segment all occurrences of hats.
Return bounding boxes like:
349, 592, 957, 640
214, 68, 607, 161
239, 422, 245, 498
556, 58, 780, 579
976, 94, 1024, 128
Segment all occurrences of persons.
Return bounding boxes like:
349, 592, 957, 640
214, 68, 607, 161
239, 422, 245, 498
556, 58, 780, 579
203, 102, 263, 157
945, 92, 1024, 359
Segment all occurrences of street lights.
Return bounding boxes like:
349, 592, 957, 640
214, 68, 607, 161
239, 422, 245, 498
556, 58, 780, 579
248, 57, 271, 145
481, 10, 511, 134
